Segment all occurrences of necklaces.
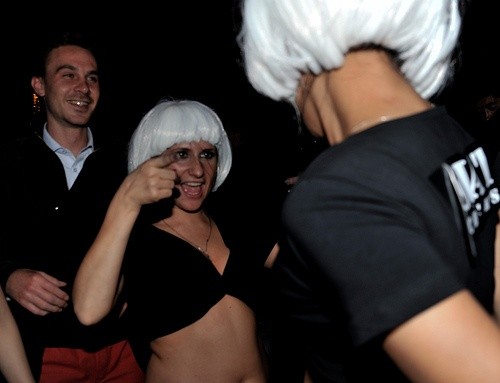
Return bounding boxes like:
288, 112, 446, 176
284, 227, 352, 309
160, 214, 212, 259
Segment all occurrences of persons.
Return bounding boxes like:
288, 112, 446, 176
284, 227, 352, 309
0, 283, 35, 383
0, 27, 146, 383
71, 98, 283, 383
473, 85, 500, 187
238, 1, 500, 383
